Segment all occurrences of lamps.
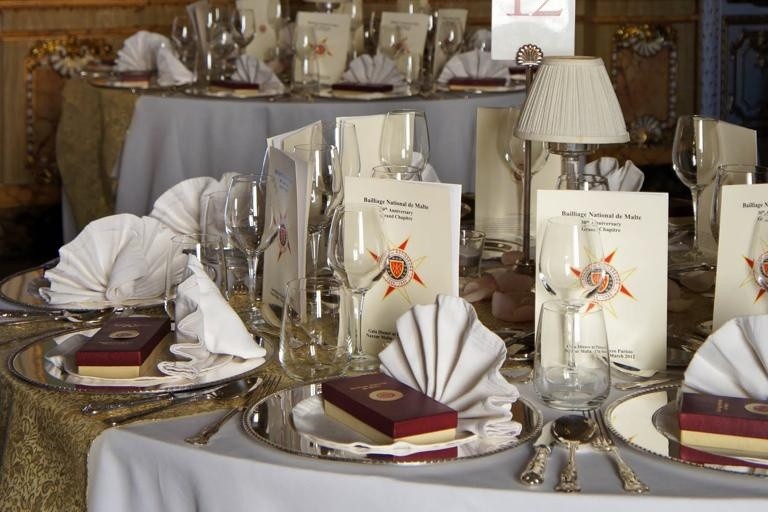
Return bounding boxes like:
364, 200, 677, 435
515, 55, 631, 192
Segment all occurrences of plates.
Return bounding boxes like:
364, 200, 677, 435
604, 382, 767, 478
240, 370, 544, 466
178, 81, 290, 100
90, 75, 186, 92
444, 77, 527, 93
7, 320, 276, 395
1, 260, 168, 311
309, 84, 421, 101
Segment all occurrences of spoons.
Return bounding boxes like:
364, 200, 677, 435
103, 376, 263, 428
0, 307, 118, 327
551, 414, 599, 494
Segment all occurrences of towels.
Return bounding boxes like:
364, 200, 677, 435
115, 30, 174, 71
379, 293, 523, 456
39, 213, 198, 309
579, 157, 645, 191
681, 314, 765, 400
341, 53, 403, 87
231, 52, 284, 88
410, 152, 442, 183
149, 170, 258, 248
156, 271, 267, 378
155, 45, 196, 88
436, 49, 511, 89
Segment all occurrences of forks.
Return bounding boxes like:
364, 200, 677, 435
582, 409, 651, 495
184, 374, 283, 446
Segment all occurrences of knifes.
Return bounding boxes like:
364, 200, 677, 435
518, 420, 557, 486
80, 381, 236, 417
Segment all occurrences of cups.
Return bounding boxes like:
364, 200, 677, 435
751, 216, 768, 290
371, 164, 423, 181
294, 27, 317, 90
224, 174, 279, 313
379, 108, 431, 181
458, 230, 486, 278
671, 114, 723, 258
163, 232, 228, 321
501, 104, 551, 176
202, 190, 237, 300
277, 276, 353, 385
534, 298, 612, 411
539, 216, 605, 387
294, 144, 344, 295
171, 7, 233, 88
556, 173, 609, 190
709, 164, 768, 247
370, 17, 415, 95
231, 8, 256, 56
310, 121, 361, 198
266, 0, 290, 57
327, 202, 390, 372
440, 21, 463, 62
